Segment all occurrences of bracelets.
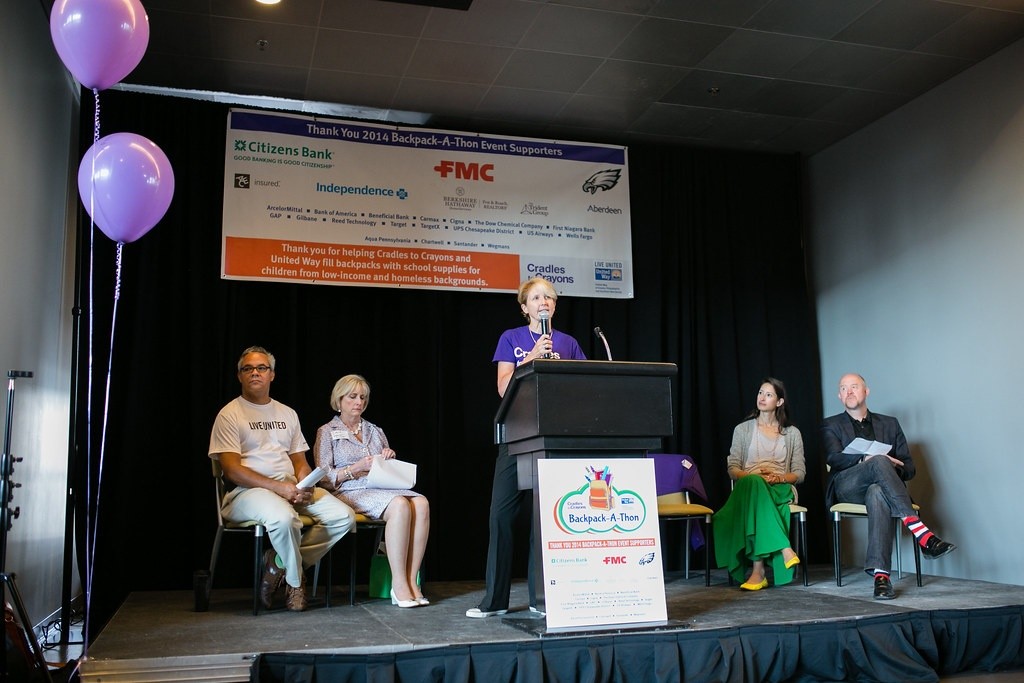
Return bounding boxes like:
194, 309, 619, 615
777, 476, 780, 484
861, 456, 865, 462
782, 475, 786, 483
344, 466, 355, 480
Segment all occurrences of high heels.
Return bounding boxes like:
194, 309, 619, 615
390, 588, 419, 607
410, 593, 429, 605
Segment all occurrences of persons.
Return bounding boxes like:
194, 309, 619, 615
313, 374, 429, 608
821, 373, 956, 600
208, 346, 356, 612
466, 276, 586, 618
713, 379, 806, 590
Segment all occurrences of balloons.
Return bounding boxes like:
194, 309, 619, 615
50, 0, 150, 92
78, 132, 175, 243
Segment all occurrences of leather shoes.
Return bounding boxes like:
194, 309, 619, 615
874, 576, 896, 599
919, 535, 957, 560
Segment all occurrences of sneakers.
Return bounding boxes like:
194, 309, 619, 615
285, 576, 309, 611
465, 605, 508, 618
530, 606, 545, 615
258, 549, 285, 609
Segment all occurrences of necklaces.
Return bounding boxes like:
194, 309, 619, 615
756, 417, 779, 467
529, 327, 552, 344
352, 419, 361, 434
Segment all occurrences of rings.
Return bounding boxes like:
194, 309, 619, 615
545, 344, 548, 348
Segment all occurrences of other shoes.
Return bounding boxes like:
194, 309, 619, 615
783, 555, 800, 569
740, 577, 769, 591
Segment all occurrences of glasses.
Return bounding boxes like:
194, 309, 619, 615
240, 366, 270, 374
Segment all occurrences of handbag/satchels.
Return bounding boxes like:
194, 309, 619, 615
369, 554, 421, 599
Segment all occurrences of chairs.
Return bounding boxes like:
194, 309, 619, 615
826, 462, 924, 588
312, 514, 427, 597
645, 454, 716, 587
203, 458, 331, 616
728, 456, 808, 587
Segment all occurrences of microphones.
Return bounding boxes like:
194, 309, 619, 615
539, 310, 551, 359
